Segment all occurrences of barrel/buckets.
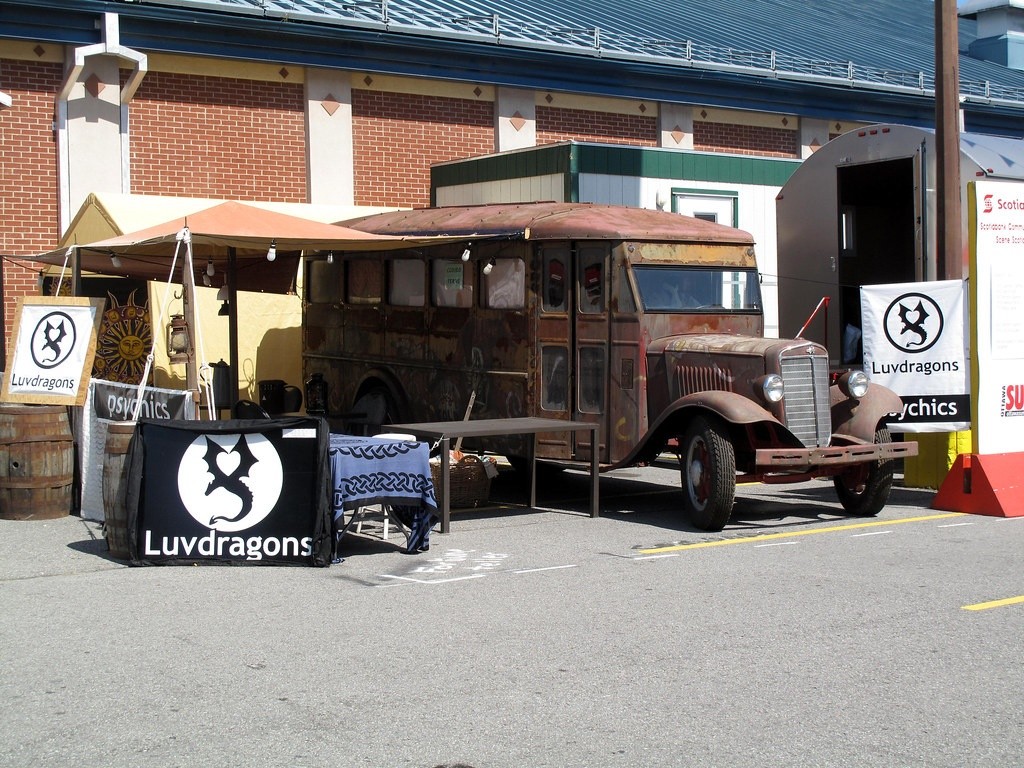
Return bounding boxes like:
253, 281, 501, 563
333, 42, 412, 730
103, 421, 140, 561
0, 403, 74, 520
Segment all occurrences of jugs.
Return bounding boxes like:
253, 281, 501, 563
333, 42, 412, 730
199, 358, 230, 404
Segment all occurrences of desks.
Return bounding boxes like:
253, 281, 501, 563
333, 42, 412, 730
329, 432, 437, 562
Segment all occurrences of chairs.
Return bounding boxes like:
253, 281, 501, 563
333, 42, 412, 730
356, 433, 417, 541
231, 400, 272, 420
266, 384, 302, 414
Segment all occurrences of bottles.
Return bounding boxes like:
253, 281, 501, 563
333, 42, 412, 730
306, 373, 327, 415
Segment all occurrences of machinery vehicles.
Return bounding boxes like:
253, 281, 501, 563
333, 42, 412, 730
302, 202, 918, 531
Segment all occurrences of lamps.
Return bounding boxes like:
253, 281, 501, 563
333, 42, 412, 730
164, 297, 196, 366
385, 417, 603, 533
216, 271, 231, 316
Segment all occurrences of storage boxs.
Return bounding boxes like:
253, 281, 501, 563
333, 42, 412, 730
903, 430, 973, 489
